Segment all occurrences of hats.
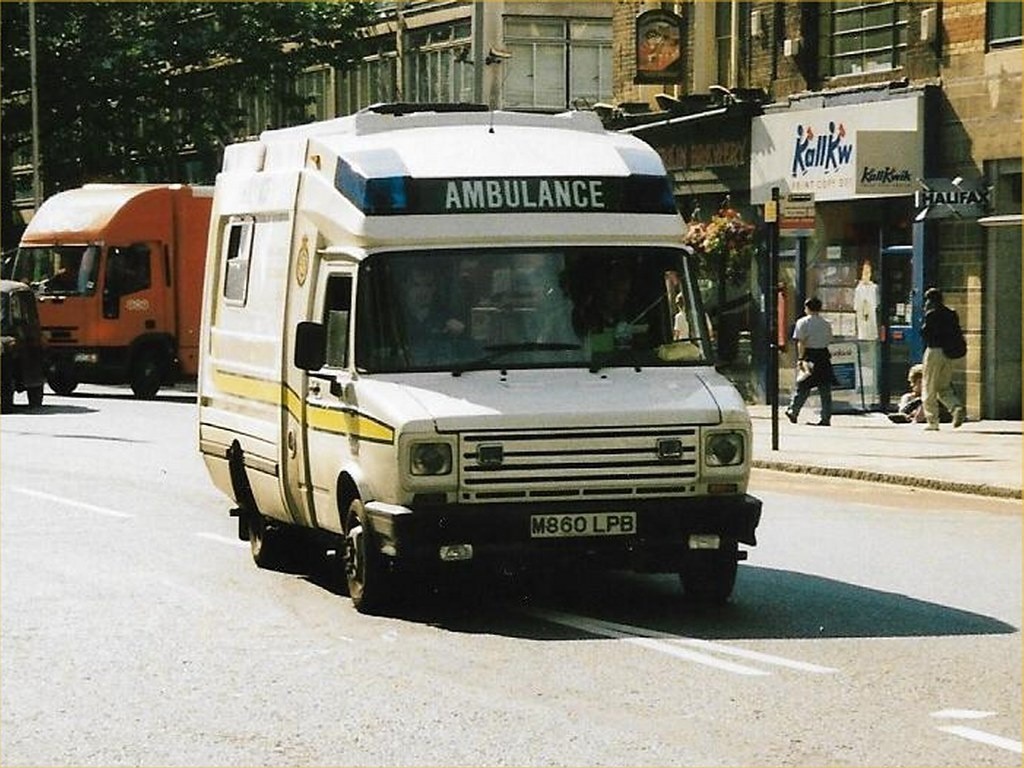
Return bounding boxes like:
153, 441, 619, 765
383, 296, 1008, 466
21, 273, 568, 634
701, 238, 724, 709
925, 288, 942, 301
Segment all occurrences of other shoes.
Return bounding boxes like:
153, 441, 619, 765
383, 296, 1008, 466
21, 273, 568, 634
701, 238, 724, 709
786, 411, 796, 423
818, 421, 830, 426
924, 427, 938, 431
953, 407, 965, 427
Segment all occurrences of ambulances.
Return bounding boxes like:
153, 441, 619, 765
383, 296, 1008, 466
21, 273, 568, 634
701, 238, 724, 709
196, 95, 763, 614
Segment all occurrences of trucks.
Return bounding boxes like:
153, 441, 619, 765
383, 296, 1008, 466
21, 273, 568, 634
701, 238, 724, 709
7, 182, 216, 401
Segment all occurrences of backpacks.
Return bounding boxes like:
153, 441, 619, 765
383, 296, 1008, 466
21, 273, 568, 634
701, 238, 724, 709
932, 311, 966, 359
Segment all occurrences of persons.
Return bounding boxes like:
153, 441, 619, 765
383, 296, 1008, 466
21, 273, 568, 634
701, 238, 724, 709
392, 260, 465, 342
784, 297, 833, 426
538, 282, 612, 344
673, 291, 713, 360
898, 288, 967, 431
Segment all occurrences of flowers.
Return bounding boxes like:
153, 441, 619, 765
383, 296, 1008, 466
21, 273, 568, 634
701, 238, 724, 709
682, 218, 708, 249
704, 209, 757, 253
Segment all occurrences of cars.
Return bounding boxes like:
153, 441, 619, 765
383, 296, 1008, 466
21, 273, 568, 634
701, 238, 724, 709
0, 280, 46, 412
